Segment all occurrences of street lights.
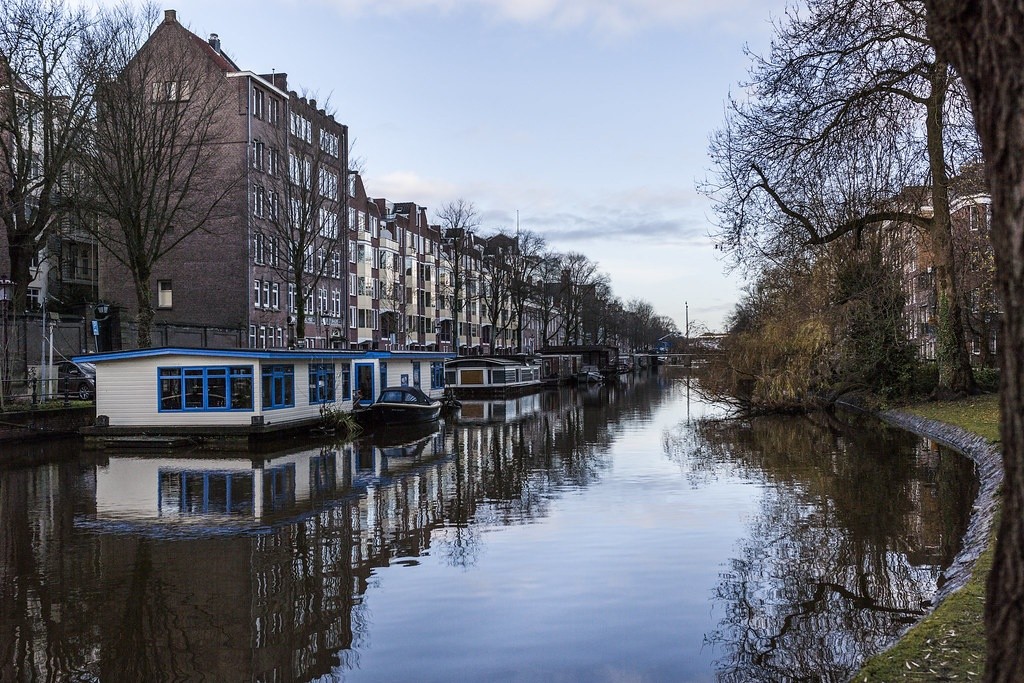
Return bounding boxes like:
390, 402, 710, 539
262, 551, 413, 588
683, 297, 690, 364
685, 365, 692, 430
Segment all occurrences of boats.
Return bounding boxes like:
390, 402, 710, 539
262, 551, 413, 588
578, 365, 602, 384
349, 383, 444, 429
352, 421, 444, 461
